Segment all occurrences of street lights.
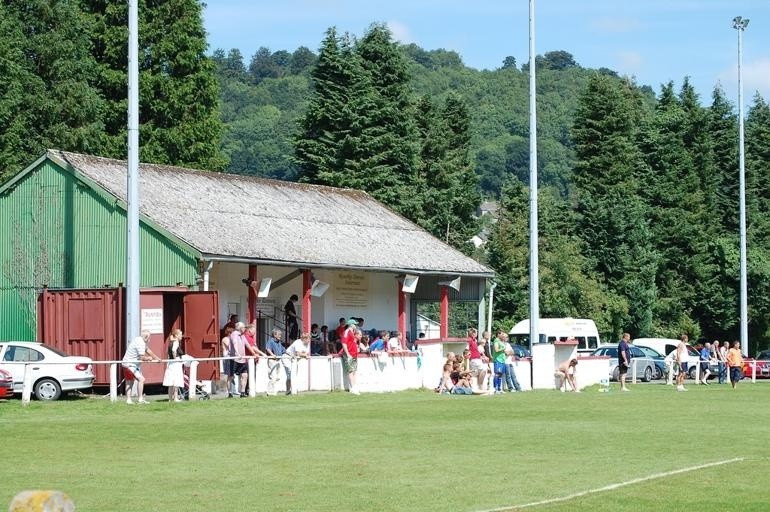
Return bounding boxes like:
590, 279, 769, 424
731, 15, 751, 360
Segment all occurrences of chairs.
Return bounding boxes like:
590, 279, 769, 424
311, 328, 411, 356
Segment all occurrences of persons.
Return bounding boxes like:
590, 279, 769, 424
220, 294, 403, 398
163, 329, 185, 401
700, 340, 743, 389
677, 334, 689, 391
434, 328, 523, 394
618, 333, 631, 391
663, 348, 678, 384
554, 359, 579, 392
121, 329, 162, 403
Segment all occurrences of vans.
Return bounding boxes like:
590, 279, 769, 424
508, 316, 600, 358
632, 337, 726, 380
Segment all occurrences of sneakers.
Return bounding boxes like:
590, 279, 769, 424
677, 388, 688, 392
559, 385, 580, 393
701, 379, 711, 386
226, 392, 249, 398
491, 388, 524, 394
349, 387, 361, 396
126, 399, 150, 406
620, 387, 630, 392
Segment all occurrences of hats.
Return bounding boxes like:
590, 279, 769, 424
348, 319, 360, 326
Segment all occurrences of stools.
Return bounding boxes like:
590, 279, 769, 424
563, 372, 573, 392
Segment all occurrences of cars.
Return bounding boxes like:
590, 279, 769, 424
0, 341, 97, 402
589, 343, 656, 383
695, 345, 770, 380
755, 350, 770, 360
635, 345, 680, 380
510, 344, 531, 357
0, 368, 14, 399
